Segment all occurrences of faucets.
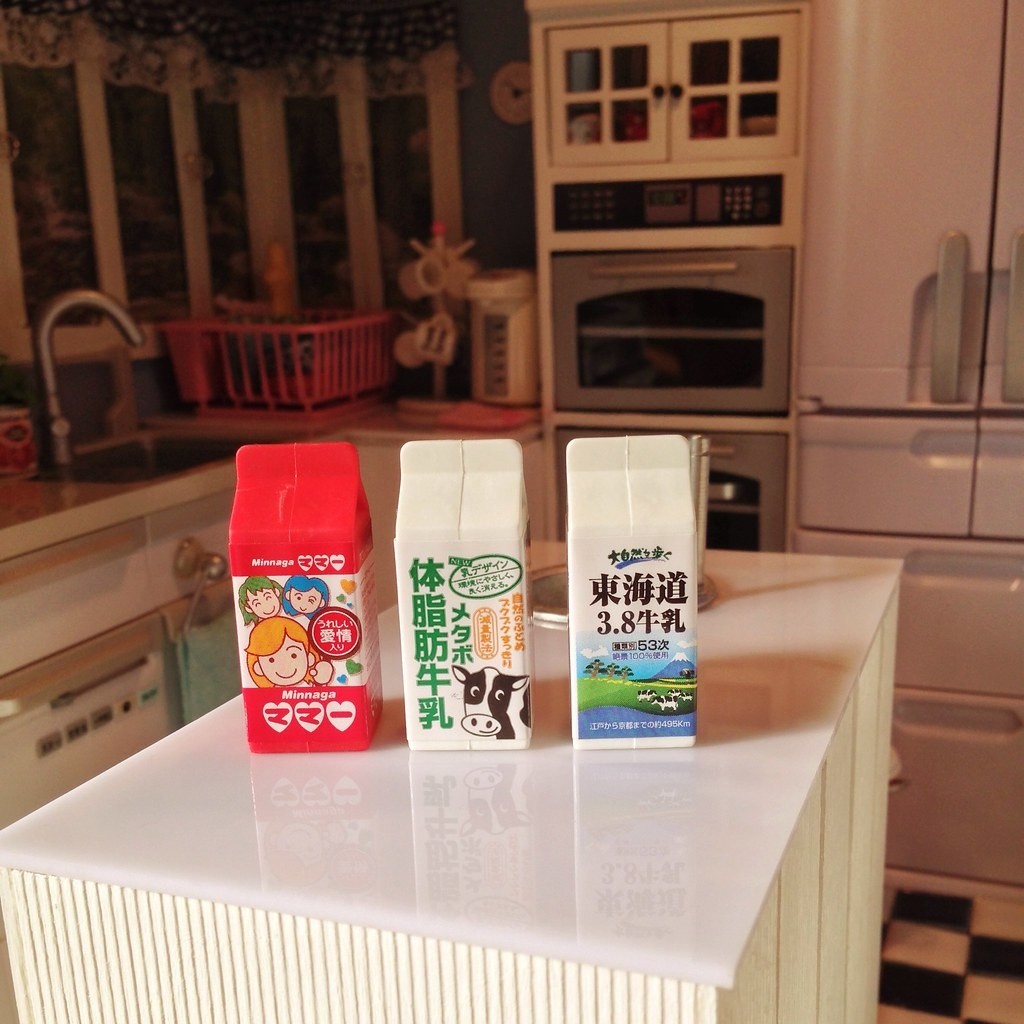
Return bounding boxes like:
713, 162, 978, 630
31, 287, 148, 469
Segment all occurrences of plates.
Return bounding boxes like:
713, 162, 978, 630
525, 563, 717, 624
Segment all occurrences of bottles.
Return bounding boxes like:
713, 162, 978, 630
0, 358, 40, 475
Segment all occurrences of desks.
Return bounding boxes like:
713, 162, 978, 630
1, 544, 906, 1024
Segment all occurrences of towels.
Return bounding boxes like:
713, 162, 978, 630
176, 607, 243, 726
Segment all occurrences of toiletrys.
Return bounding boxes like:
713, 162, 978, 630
0, 405, 35, 475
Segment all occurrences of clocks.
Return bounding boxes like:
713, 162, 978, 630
486, 60, 532, 125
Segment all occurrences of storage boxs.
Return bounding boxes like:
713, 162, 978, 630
161, 309, 402, 422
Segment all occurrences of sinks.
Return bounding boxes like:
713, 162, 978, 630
19, 433, 305, 484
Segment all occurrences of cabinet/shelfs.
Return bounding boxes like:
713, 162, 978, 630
527, 1, 802, 170
786, 0, 1024, 909
